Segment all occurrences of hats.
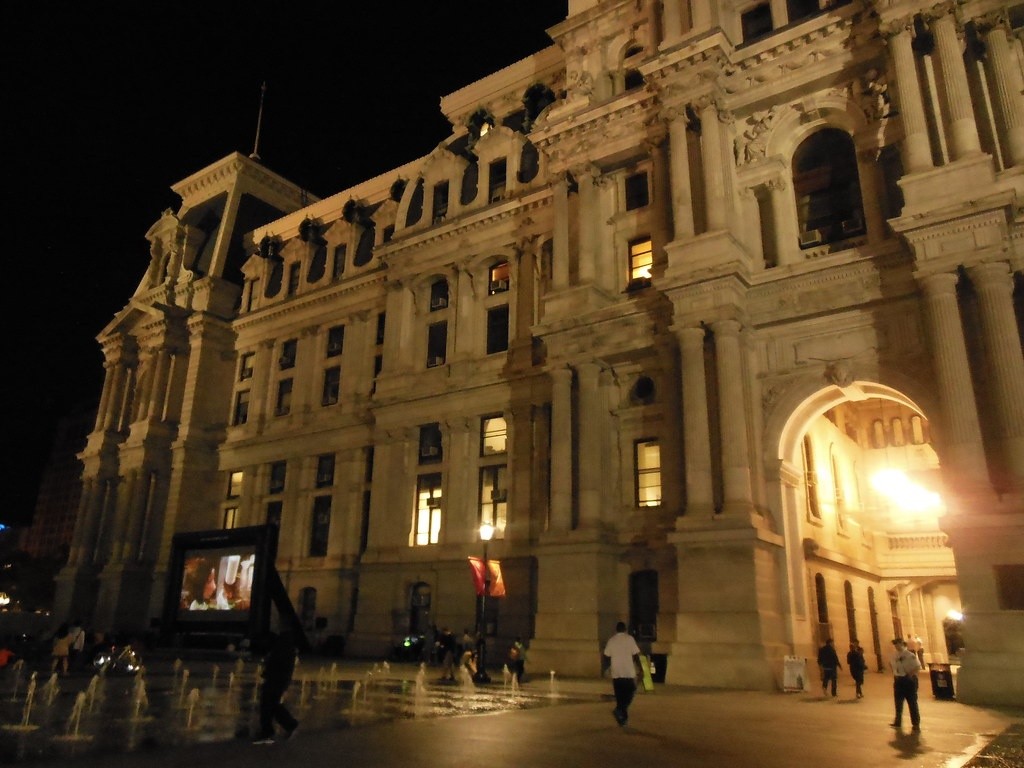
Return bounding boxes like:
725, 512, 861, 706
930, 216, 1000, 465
892, 638, 907, 646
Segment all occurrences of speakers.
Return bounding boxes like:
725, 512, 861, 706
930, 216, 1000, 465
150, 618, 161, 628
315, 616, 328, 630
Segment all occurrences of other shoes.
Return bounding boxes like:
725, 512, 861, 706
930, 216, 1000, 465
890, 723, 901, 727
857, 693, 863, 698
287, 719, 299, 740
252, 736, 274, 744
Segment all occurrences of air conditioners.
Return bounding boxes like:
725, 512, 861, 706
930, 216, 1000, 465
276, 407, 288, 416
328, 343, 341, 353
322, 397, 337, 405
434, 217, 445, 224
278, 356, 288, 365
427, 357, 444, 367
490, 489, 506, 501
421, 446, 438, 456
491, 280, 507, 291
318, 515, 329, 524
317, 471, 332, 482
237, 417, 246, 423
426, 497, 439, 507
431, 298, 446, 308
492, 193, 505, 203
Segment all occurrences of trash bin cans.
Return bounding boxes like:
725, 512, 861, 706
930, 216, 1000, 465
329, 635, 344, 659
929, 662, 956, 702
649, 653, 669, 684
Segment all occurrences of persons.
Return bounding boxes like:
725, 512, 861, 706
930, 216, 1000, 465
48, 621, 112, 680
817, 638, 843, 695
603, 624, 643, 726
847, 639, 868, 699
415, 622, 525, 684
890, 638, 921, 730
245, 571, 316, 745
0, 644, 15, 681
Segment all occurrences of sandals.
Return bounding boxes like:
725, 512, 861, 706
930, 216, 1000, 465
913, 724, 919, 730
